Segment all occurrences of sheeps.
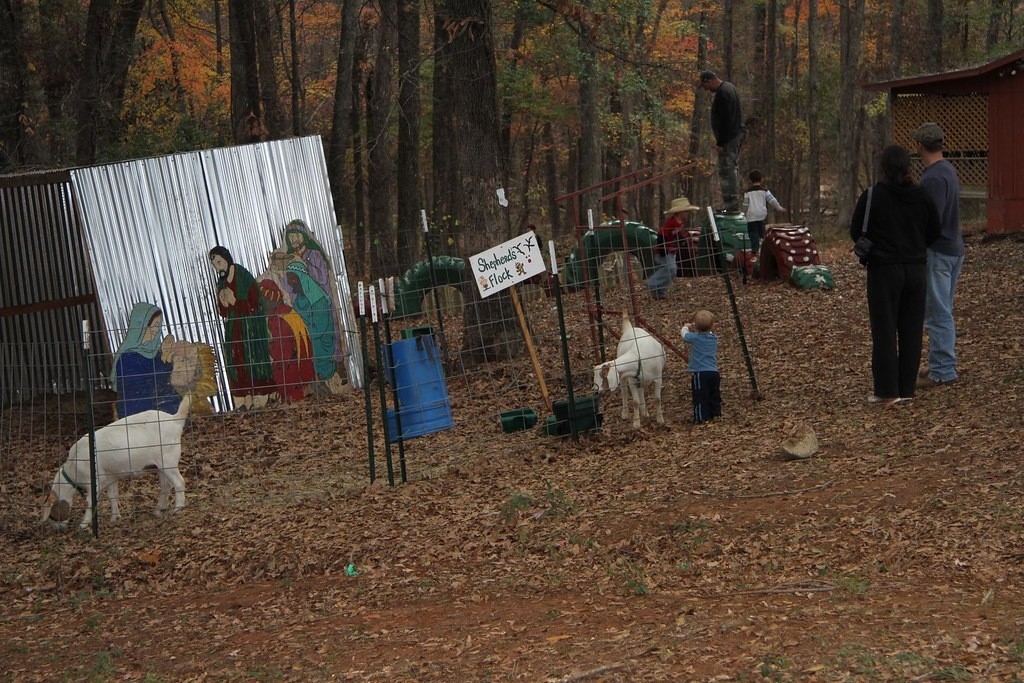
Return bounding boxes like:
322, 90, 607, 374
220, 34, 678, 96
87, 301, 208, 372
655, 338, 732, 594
591, 310, 667, 429
41, 389, 192, 534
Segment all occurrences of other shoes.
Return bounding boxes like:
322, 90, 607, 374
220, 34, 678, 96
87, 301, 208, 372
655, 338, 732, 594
649, 295, 671, 301
915, 367, 959, 389
867, 395, 912, 405
751, 250, 760, 256
720, 208, 740, 215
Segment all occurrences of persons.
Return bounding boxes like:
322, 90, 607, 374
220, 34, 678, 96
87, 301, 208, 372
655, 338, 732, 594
658, 196, 700, 275
741, 170, 786, 263
681, 310, 722, 423
913, 122, 967, 388
695, 70, 744, 216
849, 144, 943, 403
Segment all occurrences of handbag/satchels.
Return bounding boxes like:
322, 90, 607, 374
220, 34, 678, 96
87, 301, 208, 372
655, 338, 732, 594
854, 185, 873, 260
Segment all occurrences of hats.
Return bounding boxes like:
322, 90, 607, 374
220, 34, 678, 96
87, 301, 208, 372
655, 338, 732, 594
663, 197, 700, 215
697, 70, 716, 90
